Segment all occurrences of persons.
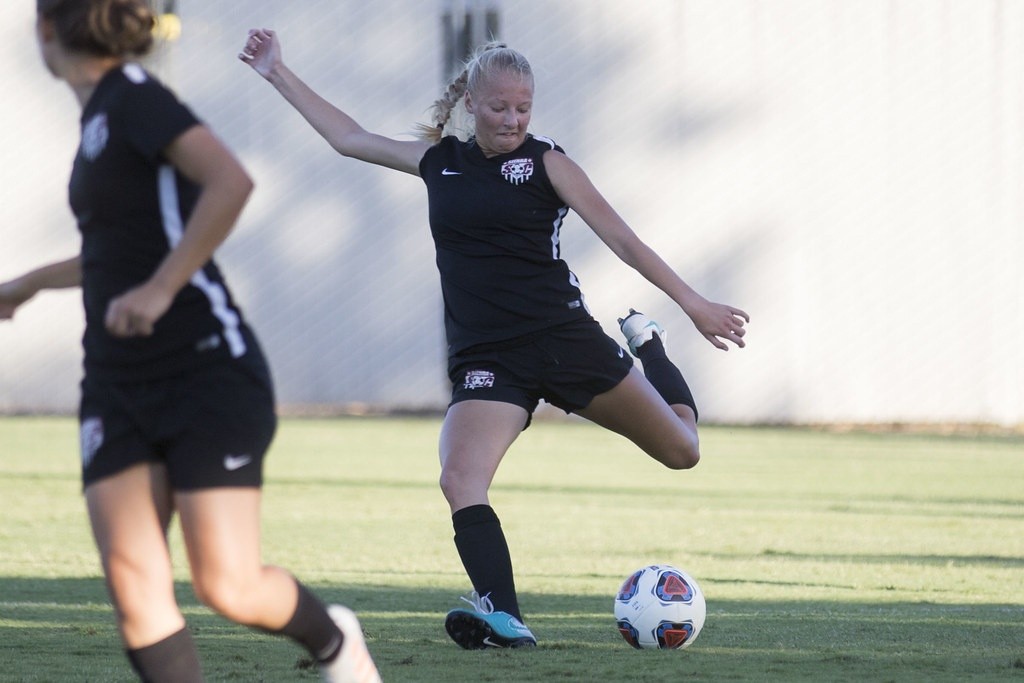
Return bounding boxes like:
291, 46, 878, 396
239, 28, 751, 650
0, 0, 383, 683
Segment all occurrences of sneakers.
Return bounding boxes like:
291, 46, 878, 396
445, 591, 536, 649
315, 604, 381, 683
618, 308, 667, 358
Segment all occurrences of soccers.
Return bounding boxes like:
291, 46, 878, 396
613, 563, 707, 651
